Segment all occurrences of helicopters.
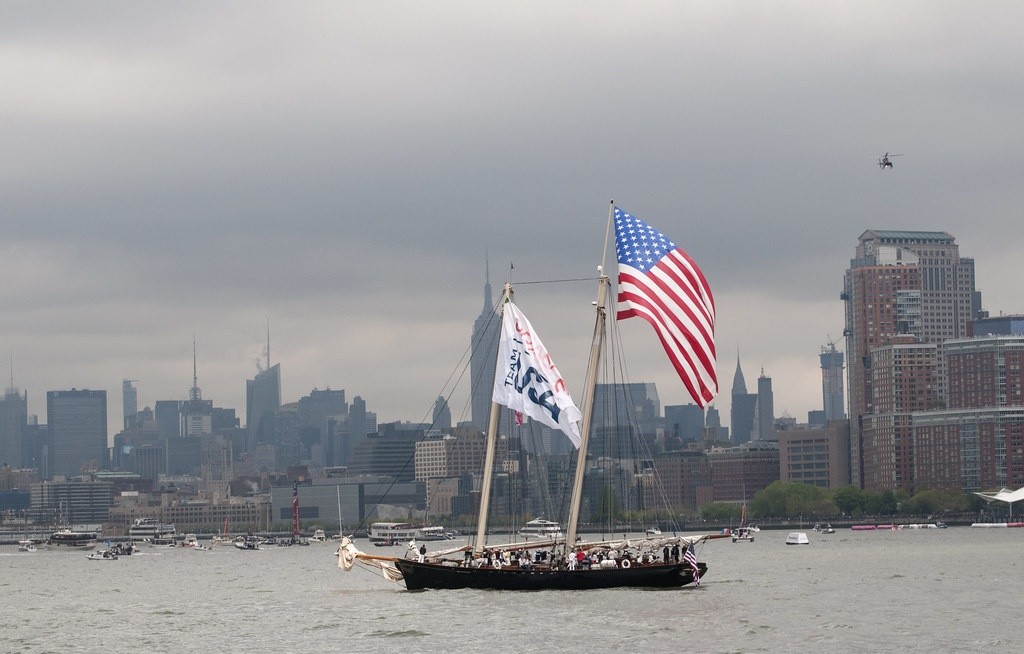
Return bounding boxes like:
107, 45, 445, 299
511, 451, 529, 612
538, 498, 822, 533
877, 152, 903, 170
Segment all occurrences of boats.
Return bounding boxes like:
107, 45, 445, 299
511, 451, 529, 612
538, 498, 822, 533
181, 518, 260, 551
518, 514, 564, 539
367, 506, 455, 548
277, 528, 292, 547
813, 522, 822, 531
786, 511, 809, 545
309, 528, 329, 545
129, 517, 177, 541
823, 522, 835, 534
645, 527, 661, 535
85, 541, 137, 560
19, 529, 97, 553
746, 522, 761, 533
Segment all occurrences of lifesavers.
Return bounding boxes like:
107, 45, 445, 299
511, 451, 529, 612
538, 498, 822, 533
493, 560, 501, 569
621, 559, 630, 568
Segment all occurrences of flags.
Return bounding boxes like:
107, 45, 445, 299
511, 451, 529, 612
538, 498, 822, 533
493, 299, 583, 450
613, 207, 719, 409
684, 541, 700, 587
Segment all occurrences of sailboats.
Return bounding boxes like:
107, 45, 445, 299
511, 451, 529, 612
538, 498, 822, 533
330, 483, 347, 543
290, 478, 311, 546
335, 200, 732, 591
732, 484, 754, 543
150, 506, 177, 546
261, 501, 276, 544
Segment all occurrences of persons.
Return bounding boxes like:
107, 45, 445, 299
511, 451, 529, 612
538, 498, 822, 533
484, 547, 560, 570
637, 551, 664, 564
563, 548, 633, 571
682, 546, 687, 557
418, 544, 426, 563
663, 546, 679, 564
